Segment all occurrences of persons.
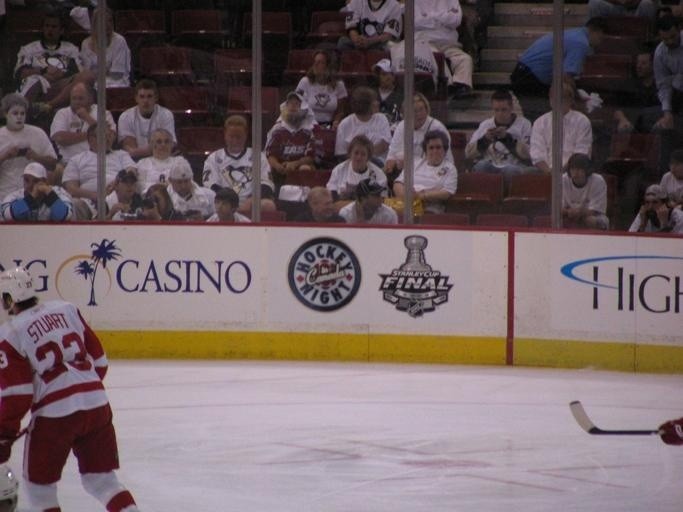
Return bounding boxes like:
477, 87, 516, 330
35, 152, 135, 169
1, 0, 681, 234
657, 416, 683, 445
1, 268, 139, 511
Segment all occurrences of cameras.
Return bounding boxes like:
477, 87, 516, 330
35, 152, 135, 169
132, 192, 158, 211
495, 131, 501, 141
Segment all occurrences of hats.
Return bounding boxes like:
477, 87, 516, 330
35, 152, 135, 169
116, 167, 137, 184
170, 156, 194, 179
370, 59, 393, 75
355, 178, 387, 196
645, 184, 667, 202
21, 162, 48, 179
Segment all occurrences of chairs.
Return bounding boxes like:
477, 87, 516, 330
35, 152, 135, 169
1, 1, 682, 229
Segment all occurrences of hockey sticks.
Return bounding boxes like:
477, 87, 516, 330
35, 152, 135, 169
570, 399, 667, 436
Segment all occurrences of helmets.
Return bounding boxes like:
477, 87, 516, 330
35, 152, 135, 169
0, 266, 36, 308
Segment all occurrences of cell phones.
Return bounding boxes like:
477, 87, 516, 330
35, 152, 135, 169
17, 148, 27, 156
